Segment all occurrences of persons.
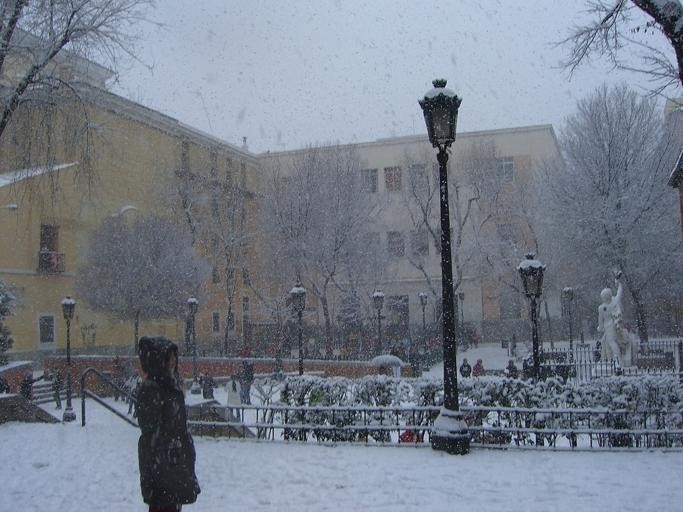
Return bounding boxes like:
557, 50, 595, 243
131, 334, 201, 511
458, 358, 471, 378
235, 364, 247, 404
50, 365, 62, 410
196, 373, 217, 400
241, 360, 254, 405
398, 342, 406, 353
472, 359, 484, 376
596, 277, 625, 363
19, 371, 46, 399
112, 373, 143, 414
397, 418, 423, 442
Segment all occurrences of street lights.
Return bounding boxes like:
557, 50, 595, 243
563, 287, 573, 363
60, 296, 76, 421
516, 253, 546, 381
374, 289, 385, 352
291, 279, 306, 376
187, 296, 202, 395
418, 293, 429, 331
419, 78, 469, 455
457, 289, 469, 349
